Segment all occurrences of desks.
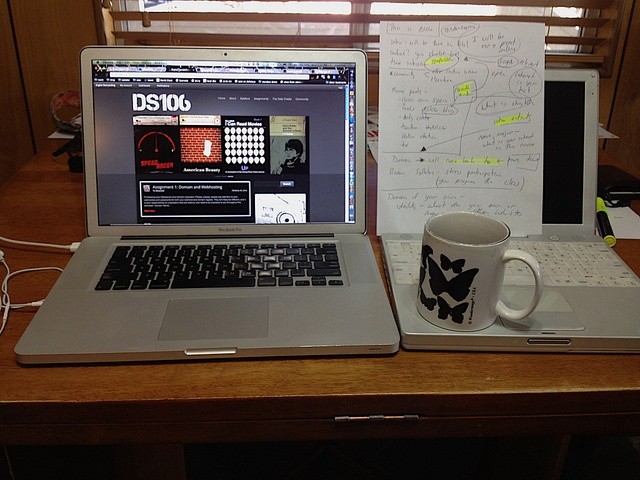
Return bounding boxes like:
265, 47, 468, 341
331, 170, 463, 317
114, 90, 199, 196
0, 137, 640, 476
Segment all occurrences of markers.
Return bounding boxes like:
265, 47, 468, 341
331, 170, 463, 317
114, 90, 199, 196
594, 196, 615, 246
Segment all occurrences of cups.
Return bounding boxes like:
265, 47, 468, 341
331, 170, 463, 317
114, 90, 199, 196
416, 212, 543, 332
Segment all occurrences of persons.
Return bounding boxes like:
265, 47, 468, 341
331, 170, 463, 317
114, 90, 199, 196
270, 139, 306, 175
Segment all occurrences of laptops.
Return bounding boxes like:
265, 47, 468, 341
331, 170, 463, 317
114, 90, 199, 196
380, 68, 640, 356
14, 45, 400, 368
